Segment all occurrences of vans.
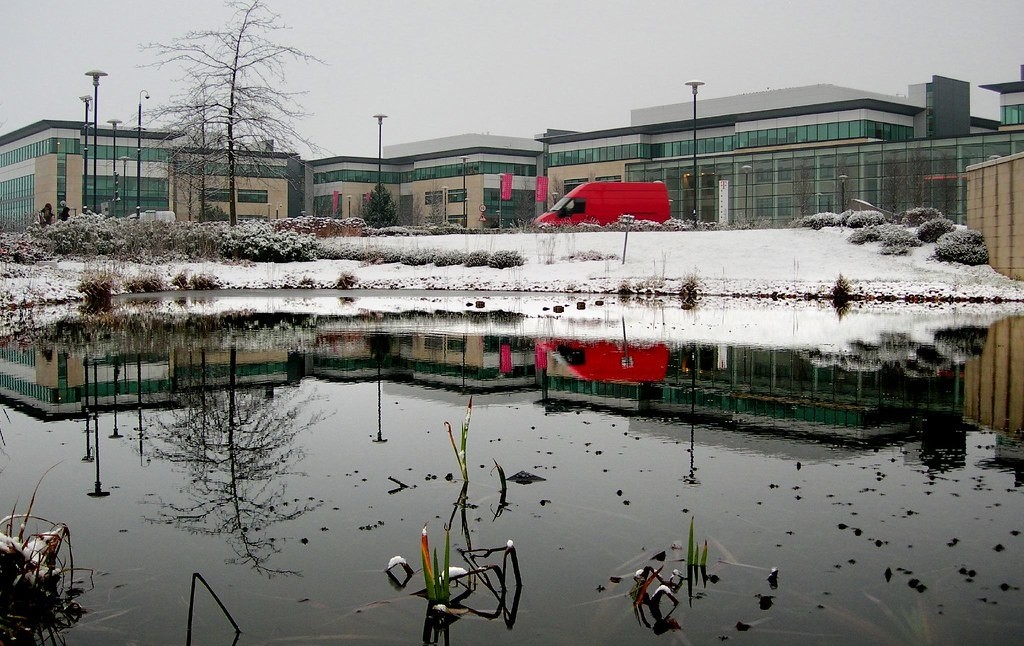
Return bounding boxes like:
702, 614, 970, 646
127, 211, 176, 224
531, 181, 671, 226
535, 337, 670, 383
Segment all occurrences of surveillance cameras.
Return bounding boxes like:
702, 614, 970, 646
145, 95, 150, 99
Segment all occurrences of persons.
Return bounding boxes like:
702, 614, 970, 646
61, 207, 70, 222
39, 203, 55, 228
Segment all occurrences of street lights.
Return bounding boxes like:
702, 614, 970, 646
373, 113, 388, 229
459, 156, 470, 228
137, 352, 152, 467
107, 119, 122, 218
686, 80, 706, 230
87, 361, 110, 499
839, 174, 847, 212
442, 186, 448, 222
80, 95, 92, 213
347, 195, 351, 218
742, 165, 752, 219
136, 89, 149, 219
685, 343, 701, 487
497, 172, 504, 229
81, 357, 96, 463
109, 352, 125, 439
118, 155, 129, 218
372, 359, 388, 444
84, 69, 108, 214
